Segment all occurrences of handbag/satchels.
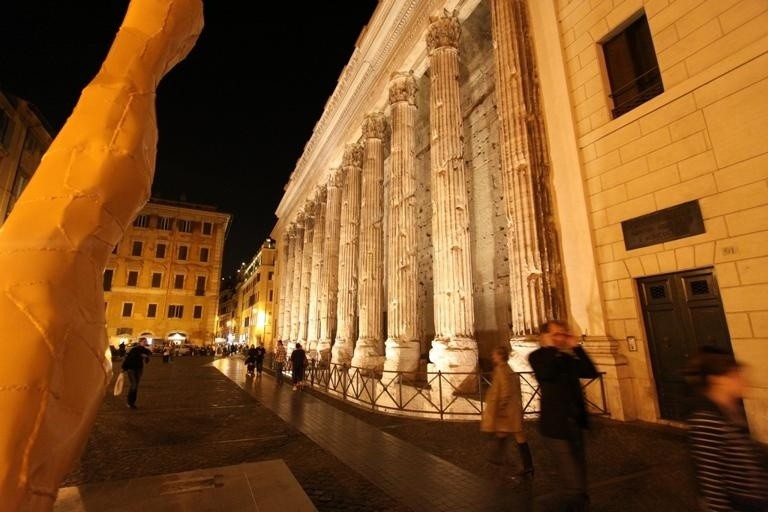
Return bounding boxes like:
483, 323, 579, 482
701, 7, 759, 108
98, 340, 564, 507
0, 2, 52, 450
114, 372, 125, 397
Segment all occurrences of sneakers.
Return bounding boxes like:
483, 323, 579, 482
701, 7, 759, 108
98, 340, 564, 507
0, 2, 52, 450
128, 404, 136, 409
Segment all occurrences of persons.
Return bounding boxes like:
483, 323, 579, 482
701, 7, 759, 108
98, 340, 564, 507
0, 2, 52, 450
272, 340, 287, 389
243, 343, 266, 377
118, 337, 152, 410
290, 342, 306, 392
475, 342, 534, 481
113, 335, 243, 363
525, 320, 600, 512
681, 351, 768, 512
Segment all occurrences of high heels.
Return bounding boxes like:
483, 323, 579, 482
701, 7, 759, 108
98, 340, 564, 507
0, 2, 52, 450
519, 468, 535, 479
